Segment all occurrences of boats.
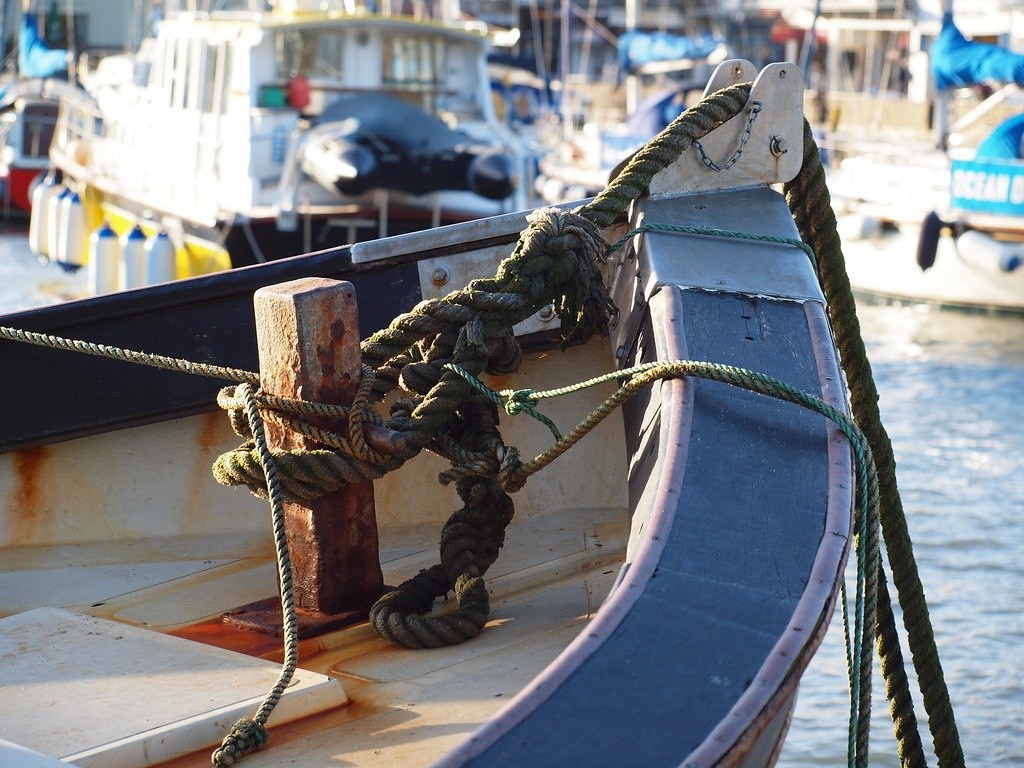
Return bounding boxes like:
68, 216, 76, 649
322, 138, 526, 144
0, 64, 852, 768
486, 0, 1024, 319
25, 0, 544, 303
0, 0, 133, 231
